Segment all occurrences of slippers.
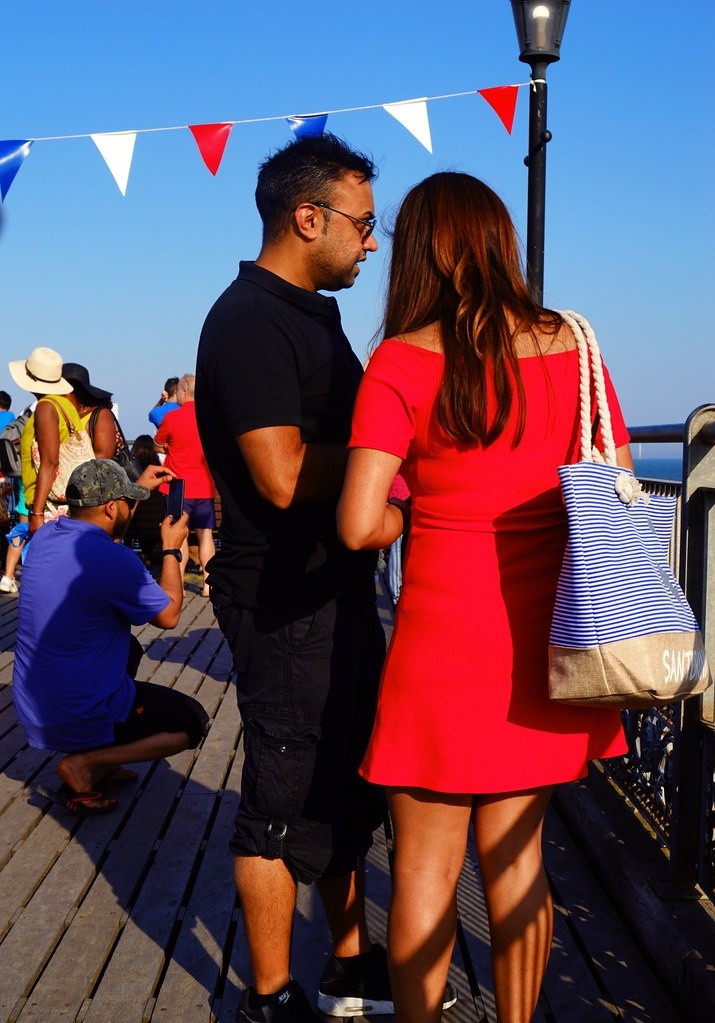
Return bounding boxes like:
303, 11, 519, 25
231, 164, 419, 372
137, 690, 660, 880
35, 785, 119, 814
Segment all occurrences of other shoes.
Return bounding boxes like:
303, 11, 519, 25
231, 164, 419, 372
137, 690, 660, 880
192, 563, 202, 572
0, 575, 19, 594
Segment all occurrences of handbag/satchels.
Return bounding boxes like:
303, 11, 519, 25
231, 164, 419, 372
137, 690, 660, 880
89, 406, 141, 483
549, 309, 713, 705
7, 523, 33, 565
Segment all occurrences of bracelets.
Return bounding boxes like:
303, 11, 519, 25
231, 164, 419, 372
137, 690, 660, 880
31, 511, 44, 516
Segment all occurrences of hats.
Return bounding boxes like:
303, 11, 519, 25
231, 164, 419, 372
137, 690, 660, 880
62, 363, 114, 398
66, 458, 150, 507
9, 347, 74, 395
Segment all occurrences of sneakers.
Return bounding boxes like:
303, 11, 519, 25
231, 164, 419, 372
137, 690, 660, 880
237, 978, 325, 1023
318, 941, 457, 1017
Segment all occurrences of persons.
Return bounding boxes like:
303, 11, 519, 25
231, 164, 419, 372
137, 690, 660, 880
0, 346, 138, 593
130, 375, 218, 597
340, 172, 635, 1023
195, 134, 457, 1023
11, 459, 209, 816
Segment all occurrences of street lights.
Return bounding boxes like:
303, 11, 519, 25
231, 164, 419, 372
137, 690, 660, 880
510, 0, 571, 308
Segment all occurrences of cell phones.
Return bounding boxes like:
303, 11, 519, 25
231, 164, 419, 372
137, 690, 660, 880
166, 479, 184, 525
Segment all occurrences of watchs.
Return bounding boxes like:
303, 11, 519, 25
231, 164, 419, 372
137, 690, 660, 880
162, 549, 182, 562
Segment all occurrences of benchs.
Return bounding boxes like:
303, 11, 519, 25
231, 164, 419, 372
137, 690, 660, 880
122, 494, 223, 550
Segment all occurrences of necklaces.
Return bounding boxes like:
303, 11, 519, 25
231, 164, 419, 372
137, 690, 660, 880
77, 407, 89, 414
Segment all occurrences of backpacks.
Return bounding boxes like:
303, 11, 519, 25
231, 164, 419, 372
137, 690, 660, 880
1, 402, 34, 478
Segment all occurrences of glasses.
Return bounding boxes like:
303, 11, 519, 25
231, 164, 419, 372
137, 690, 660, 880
312, 203, 378, 239
